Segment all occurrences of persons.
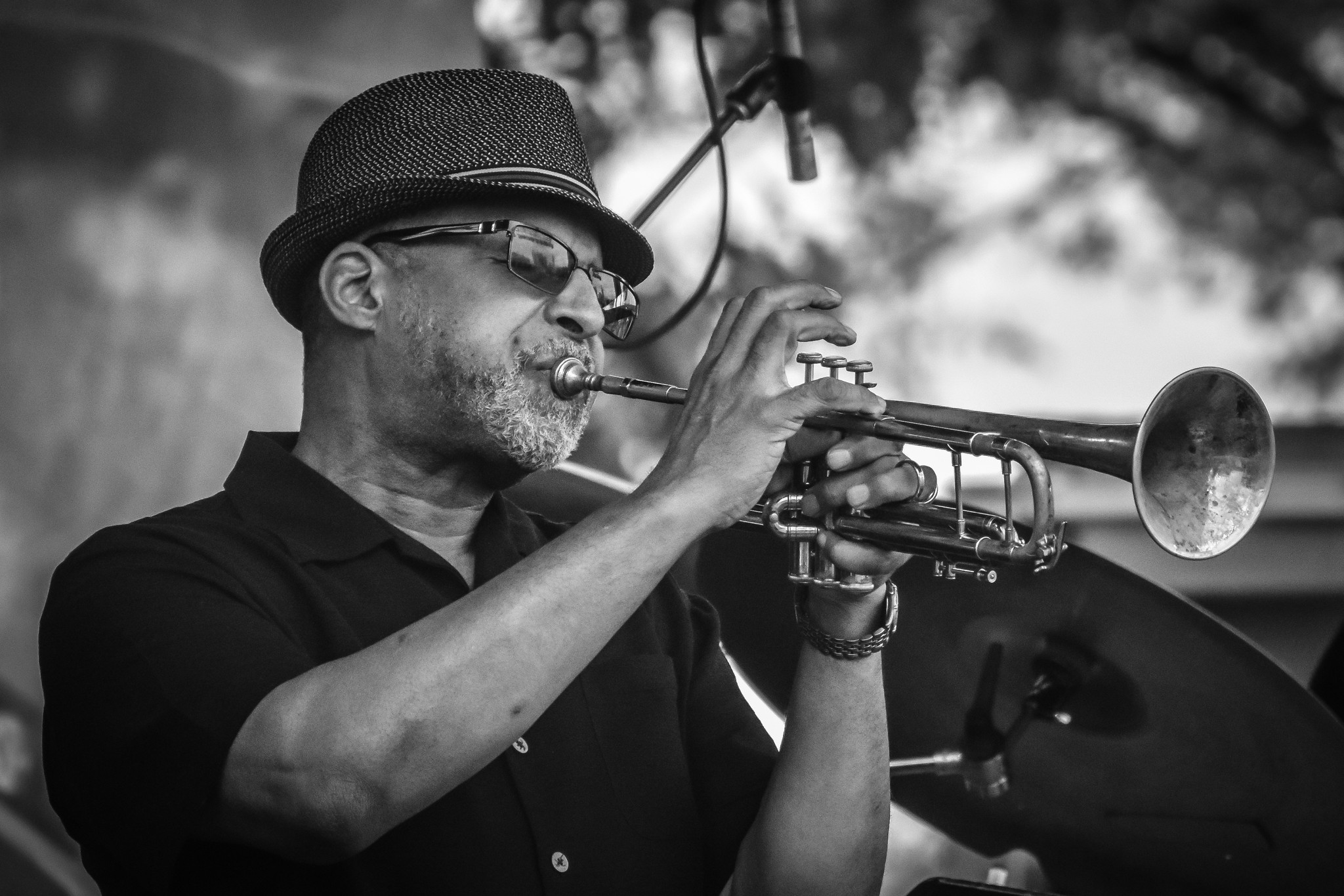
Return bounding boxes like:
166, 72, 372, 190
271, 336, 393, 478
40, 69, 935, 896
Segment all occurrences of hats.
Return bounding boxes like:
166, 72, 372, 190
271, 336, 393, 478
262, 66, 657, 327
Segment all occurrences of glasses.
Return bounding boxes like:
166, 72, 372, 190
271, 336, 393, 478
361, 217, 640, 341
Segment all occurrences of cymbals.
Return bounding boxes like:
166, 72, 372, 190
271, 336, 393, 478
699, 494, 1344, 895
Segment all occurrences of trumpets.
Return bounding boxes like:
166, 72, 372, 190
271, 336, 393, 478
547, 342, 1277, 591
